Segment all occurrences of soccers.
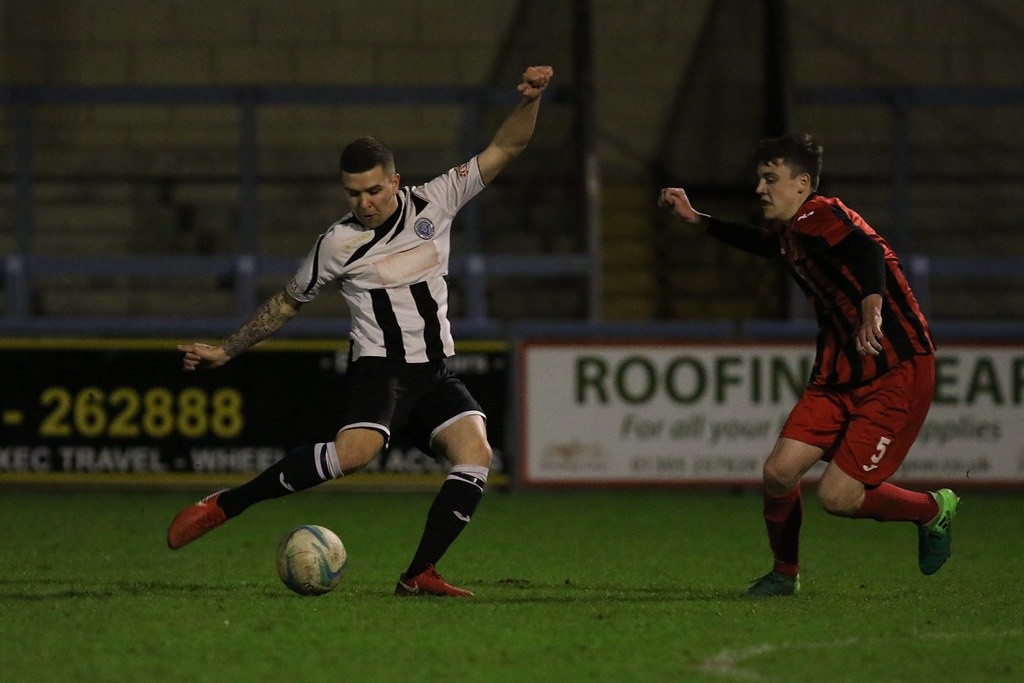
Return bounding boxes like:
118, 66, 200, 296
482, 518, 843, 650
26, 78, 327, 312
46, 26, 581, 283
275, 526, 350, 596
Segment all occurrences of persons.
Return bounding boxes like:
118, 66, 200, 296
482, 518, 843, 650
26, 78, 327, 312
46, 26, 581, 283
656, 134, 960, 599
166, 65, 553, 597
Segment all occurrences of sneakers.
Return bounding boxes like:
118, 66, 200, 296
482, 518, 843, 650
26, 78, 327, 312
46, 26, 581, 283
745, 572, 798, 596
914, 489, 960, 575
394, 565, 473, 597
167, 489, 230, 551
